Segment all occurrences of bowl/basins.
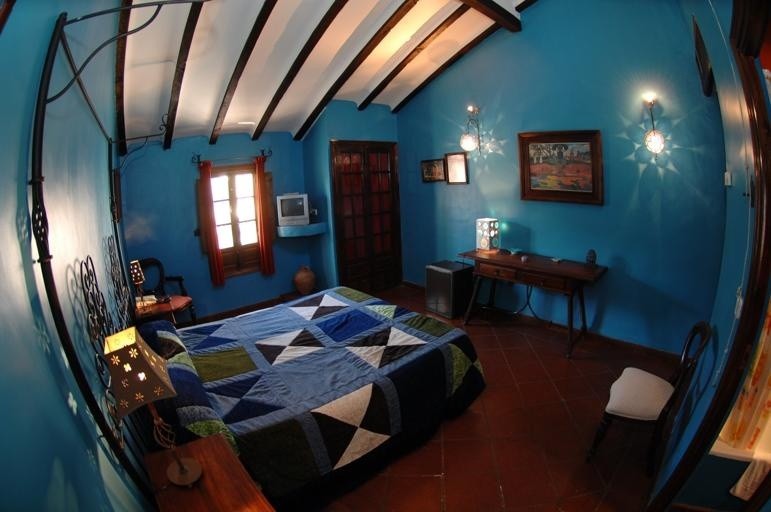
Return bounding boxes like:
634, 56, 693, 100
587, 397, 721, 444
507, 247, 524, 255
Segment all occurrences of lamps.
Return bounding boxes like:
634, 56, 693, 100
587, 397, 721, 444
102, 322, 206, 490
129, 259, 150, 312
457, 102, 485, 156
642, 90, 666, 157
475, 216, 500, 255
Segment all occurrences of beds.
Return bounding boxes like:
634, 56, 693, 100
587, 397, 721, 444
65, 234, 488, 511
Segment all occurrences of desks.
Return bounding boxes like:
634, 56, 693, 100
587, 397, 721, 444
141, 432, 279, 512
457, 248, 607, 362
131, 294, 194, 329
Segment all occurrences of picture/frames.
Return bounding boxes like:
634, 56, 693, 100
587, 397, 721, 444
420, 157, 446, 183
443, 151, 470, 187
514, 128, 606, 208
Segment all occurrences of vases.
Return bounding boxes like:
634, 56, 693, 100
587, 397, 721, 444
293, 266, 318, 297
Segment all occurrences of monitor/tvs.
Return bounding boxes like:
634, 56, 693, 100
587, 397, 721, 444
277, 194, 309, 226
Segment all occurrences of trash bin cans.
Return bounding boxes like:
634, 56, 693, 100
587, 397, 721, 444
424, 260, 475, 320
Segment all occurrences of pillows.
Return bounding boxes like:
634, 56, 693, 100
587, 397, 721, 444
134, 319, 240, 460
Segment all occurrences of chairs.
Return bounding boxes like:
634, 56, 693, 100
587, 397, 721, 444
580, 319, 715, 477
133, 256, 199, 329
